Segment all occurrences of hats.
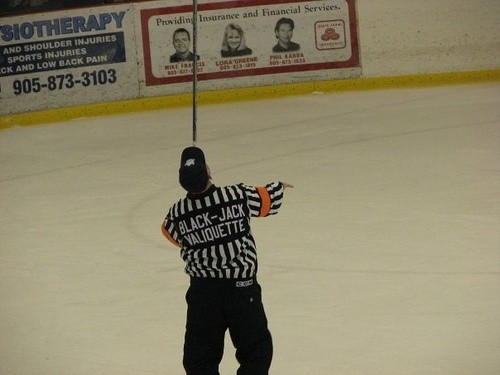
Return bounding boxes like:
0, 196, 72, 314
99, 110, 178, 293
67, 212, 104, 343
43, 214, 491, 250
179, 146, 208, 193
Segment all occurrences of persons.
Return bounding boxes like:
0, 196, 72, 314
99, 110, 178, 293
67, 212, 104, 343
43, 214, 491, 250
220, 21, 253, 58
170, 28, 200, 63
272, 18, 301, 52
161, 147, 295, 375
